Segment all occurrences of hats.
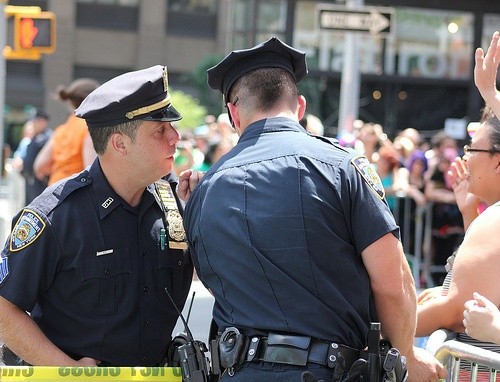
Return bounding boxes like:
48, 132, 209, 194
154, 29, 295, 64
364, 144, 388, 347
74, 65, 183, 124
207, 37, 308, 105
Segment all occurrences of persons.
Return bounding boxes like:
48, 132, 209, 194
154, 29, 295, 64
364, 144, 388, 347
0, 64, 208, 381
473, 30, 500, 120
184, 36, 449, 382
463, 292, 499, 347
9, 121, 33, 169
170, 105, 463, 287
20, 114, 51, 207
32, 76, 100, 188
414, 117, 500, 382
447, 156, 478, 233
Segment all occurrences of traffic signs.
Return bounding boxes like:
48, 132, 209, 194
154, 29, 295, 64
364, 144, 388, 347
313, 5, 397, 38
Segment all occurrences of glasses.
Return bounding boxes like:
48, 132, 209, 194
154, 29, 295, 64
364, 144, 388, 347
463, 144, 499, 156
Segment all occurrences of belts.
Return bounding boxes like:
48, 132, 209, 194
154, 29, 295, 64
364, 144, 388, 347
213, 325, 361, 372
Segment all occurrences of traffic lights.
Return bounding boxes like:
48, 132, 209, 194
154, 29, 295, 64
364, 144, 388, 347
3, 5, 59, 62
